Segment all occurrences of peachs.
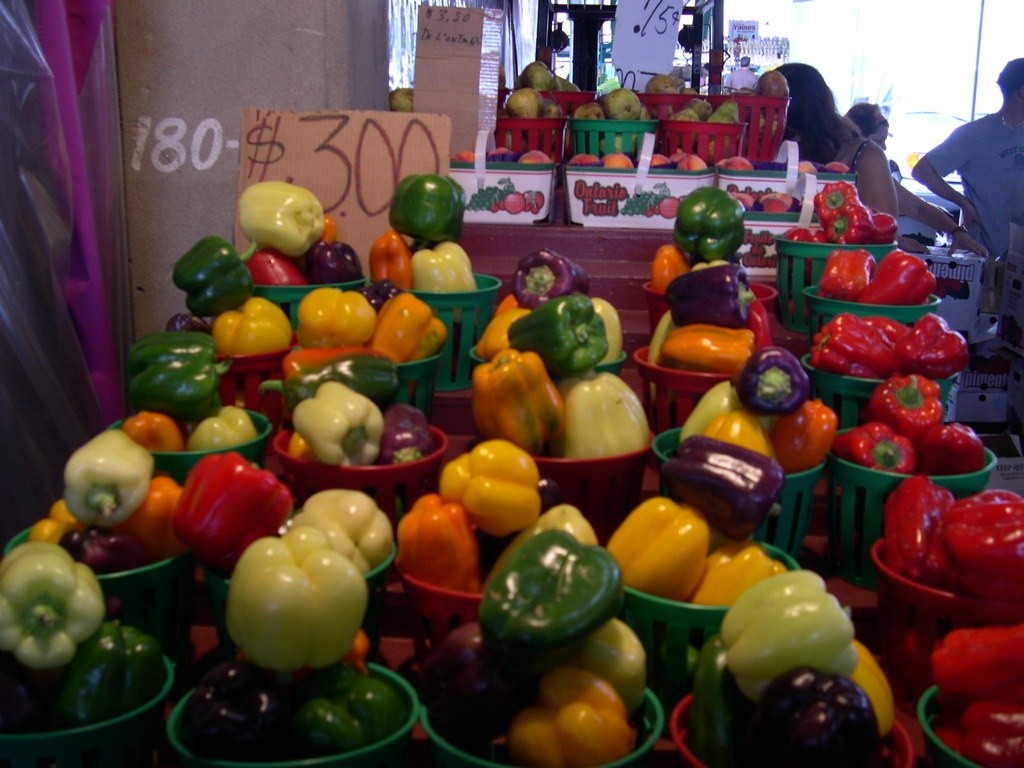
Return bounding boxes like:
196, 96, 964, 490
453, 147, 852, 214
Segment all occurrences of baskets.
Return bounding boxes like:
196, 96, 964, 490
0, 88, 1024, 768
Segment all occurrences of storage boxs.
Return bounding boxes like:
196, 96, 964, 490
448, 160, 857, 281
895, 196, 1024, 496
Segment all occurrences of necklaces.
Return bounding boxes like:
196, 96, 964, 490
998, 113, 1024, 132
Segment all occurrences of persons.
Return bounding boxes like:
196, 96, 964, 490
843, 103, 988, 259
725, 56, 758, 95
773, 63, 899, 223
911, 58, 1024, 260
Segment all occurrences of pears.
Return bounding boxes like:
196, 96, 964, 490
389, 61, 789, 160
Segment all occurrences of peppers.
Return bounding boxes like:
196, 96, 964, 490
0, 175, 1024, 768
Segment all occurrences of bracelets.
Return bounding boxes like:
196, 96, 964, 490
949, 226, 967, 237
